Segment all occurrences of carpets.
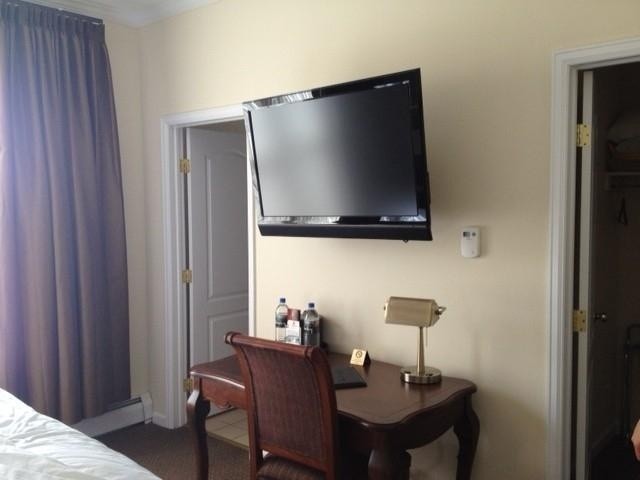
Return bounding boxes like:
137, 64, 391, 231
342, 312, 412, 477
93, 425, 249, 480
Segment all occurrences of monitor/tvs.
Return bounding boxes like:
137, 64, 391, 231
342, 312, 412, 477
242, 67, 435, 241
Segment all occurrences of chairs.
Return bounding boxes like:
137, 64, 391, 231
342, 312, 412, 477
224, 333, 412, 480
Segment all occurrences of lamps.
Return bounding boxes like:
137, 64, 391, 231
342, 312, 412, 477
383, 295, 447, 385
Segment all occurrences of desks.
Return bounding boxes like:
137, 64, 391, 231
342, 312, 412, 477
186, 351, 479, 480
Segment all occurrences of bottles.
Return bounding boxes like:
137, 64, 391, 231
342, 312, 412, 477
274, 298, 321, 344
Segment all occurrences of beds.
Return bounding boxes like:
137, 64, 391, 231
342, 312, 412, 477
0, 388, 163, 480
